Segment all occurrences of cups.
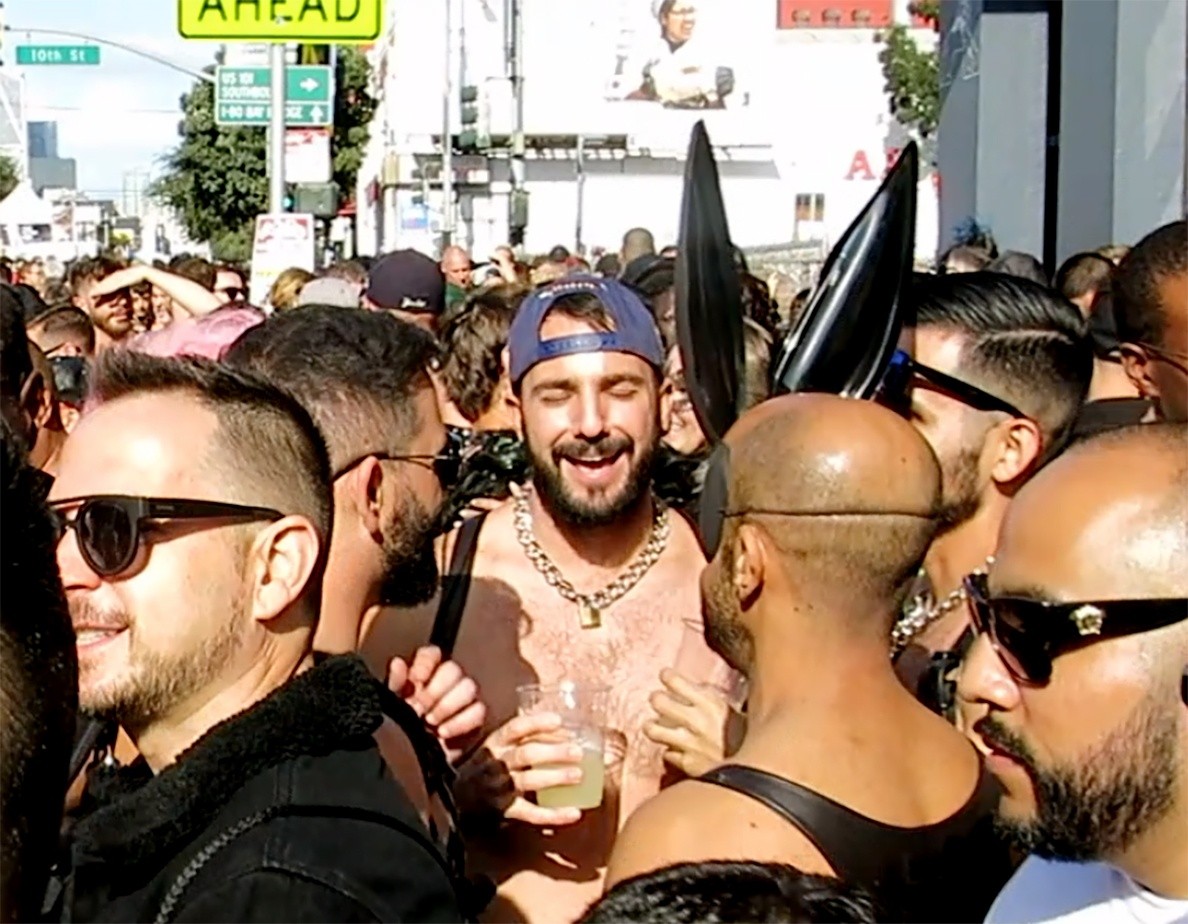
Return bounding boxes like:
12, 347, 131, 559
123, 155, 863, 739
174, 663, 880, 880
657, 618, 741, 727
515, 680, 609, 813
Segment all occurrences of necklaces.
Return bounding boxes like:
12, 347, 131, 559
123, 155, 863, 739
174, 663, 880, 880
888, 554, 994, 662
510, 486, 669, 628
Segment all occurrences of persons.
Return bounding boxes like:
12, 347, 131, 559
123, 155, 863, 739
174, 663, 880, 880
0, 225, 1188, 924
625, 0, 726, 110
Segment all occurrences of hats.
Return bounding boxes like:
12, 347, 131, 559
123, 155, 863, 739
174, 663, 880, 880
508, 276, 667, 393
365, 248, 448, 314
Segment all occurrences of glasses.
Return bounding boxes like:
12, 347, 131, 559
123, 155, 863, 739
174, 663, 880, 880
882, 348, 1029, 422
214, 287, 250, 301
330, 433, 462, 494
23, 495, 290, 577
670, 8, 694, 15
958, 570, 1186, 687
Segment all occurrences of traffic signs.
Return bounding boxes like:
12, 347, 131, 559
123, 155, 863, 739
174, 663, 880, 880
214, 64, 334, 127
